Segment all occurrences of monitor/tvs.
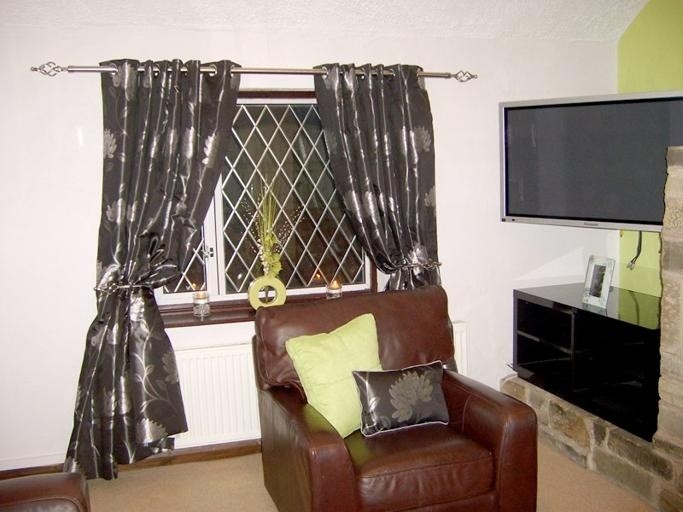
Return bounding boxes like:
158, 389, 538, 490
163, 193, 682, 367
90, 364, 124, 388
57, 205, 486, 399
498, 89, 683, 233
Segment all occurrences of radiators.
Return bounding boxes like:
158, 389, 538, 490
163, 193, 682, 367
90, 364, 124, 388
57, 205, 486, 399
160, 321, 470, 455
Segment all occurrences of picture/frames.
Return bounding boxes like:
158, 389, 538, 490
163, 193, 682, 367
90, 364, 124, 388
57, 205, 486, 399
581, 254, 615, 311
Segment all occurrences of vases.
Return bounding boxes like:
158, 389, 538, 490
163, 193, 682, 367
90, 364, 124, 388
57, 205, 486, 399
247, 277, 288, 311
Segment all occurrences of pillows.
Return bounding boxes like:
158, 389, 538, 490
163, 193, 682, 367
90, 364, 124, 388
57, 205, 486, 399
281, 310, 387, 441
355, 359, 450, 439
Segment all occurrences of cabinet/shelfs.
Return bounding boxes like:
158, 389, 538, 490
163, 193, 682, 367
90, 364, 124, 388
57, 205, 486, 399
511, 282, 660, 444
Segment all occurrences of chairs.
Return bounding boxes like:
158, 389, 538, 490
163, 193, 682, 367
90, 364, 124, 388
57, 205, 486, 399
0, 469, 89, 512
252, 283, 537, 509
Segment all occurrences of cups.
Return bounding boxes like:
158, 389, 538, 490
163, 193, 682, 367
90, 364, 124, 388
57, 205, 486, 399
326, 286, 342, 300
193, 293, 210, 319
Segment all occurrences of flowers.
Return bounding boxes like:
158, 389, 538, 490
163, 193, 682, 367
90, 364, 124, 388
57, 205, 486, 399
238, 173, 308, 301
